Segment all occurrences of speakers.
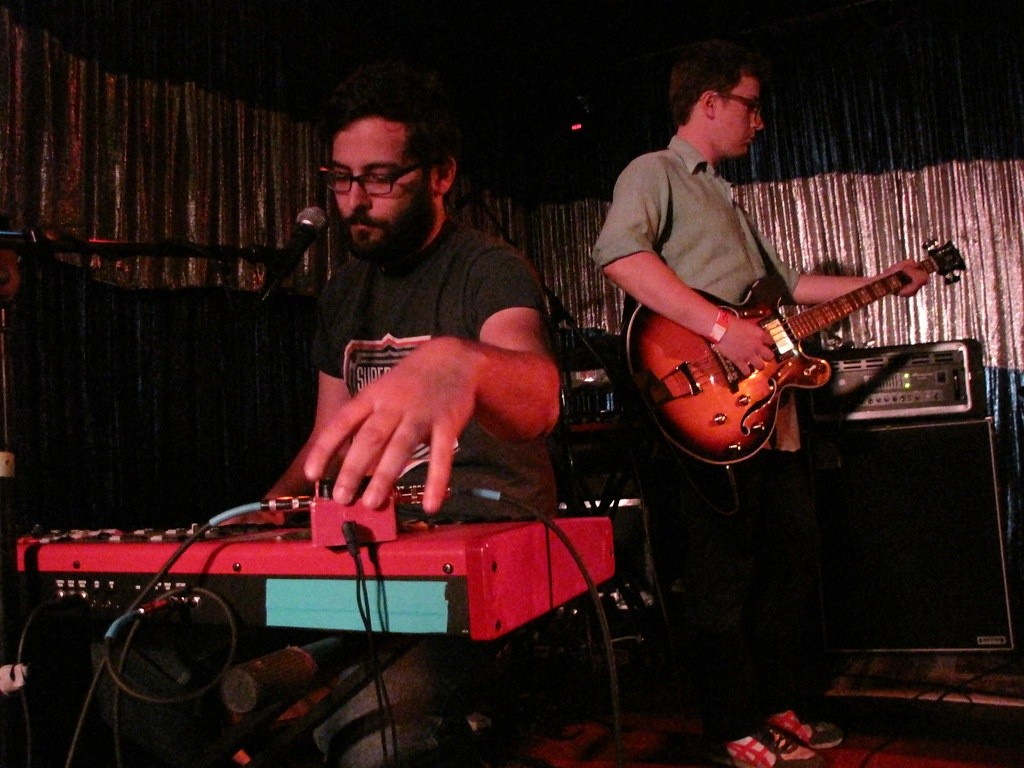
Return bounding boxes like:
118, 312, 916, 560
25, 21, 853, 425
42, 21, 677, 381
811, 416, 1016, 653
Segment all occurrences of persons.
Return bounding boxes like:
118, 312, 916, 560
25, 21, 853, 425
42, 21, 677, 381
251, 57, 568, 767
591, 43, 929, 768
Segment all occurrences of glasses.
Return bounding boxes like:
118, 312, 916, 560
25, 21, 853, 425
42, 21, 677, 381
318, 156, 433, 196
694, 90, 762, 121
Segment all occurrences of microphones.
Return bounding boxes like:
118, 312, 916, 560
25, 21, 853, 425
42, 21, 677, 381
256, 206, 327, 304
471, 203, 483, 228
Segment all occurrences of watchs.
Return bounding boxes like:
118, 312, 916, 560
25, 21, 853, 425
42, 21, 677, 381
710, 307, 728, 344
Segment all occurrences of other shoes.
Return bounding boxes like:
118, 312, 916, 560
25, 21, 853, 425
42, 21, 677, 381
765, 705, 844, 749
708, 726, 826, 768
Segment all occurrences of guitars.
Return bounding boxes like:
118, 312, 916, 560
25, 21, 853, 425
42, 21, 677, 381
626, 236, 969, 468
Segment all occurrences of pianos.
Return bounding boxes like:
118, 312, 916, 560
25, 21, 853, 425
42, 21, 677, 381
15, 513, 613, 641
